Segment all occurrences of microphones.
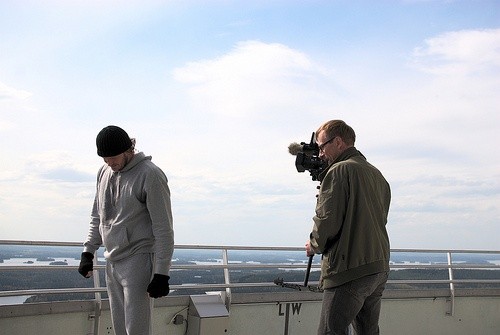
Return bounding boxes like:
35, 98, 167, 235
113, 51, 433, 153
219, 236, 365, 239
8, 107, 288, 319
288, 142, 304, 155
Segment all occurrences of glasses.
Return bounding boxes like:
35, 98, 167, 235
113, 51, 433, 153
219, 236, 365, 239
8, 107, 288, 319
318, 135, 338, 150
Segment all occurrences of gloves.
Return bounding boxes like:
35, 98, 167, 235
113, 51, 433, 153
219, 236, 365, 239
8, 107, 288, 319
147, 274, 171, 299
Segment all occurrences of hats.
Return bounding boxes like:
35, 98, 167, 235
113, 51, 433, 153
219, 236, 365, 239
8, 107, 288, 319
96, 125, 132, 157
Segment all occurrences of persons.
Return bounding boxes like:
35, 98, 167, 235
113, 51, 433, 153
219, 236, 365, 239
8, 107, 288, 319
78, 125, 175, 335
306, 120, 391, 334
